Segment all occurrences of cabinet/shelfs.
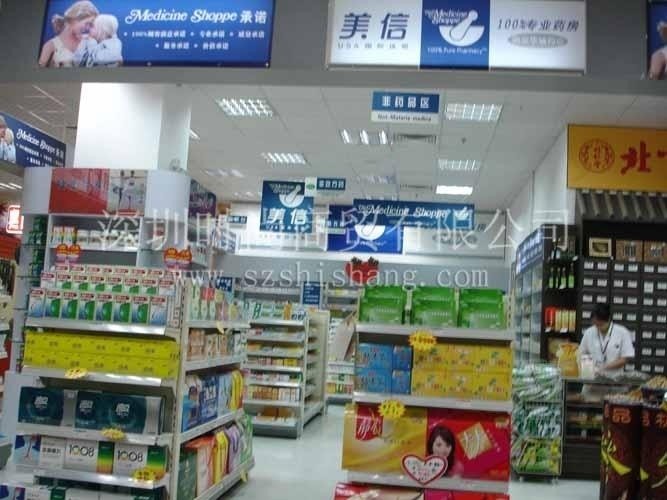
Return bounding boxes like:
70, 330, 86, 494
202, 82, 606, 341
512, 258, 666, 487
3, 276, 331, 499
341, 321, 511, 494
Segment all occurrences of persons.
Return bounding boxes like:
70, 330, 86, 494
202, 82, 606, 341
574, 303, 636, 399
647, 20, 667, 80
428, 424, 465, 479
0, 115, 8, 161
4, 128, 16, 164
38, 0, 100, 67
88, 14, 123, 67
